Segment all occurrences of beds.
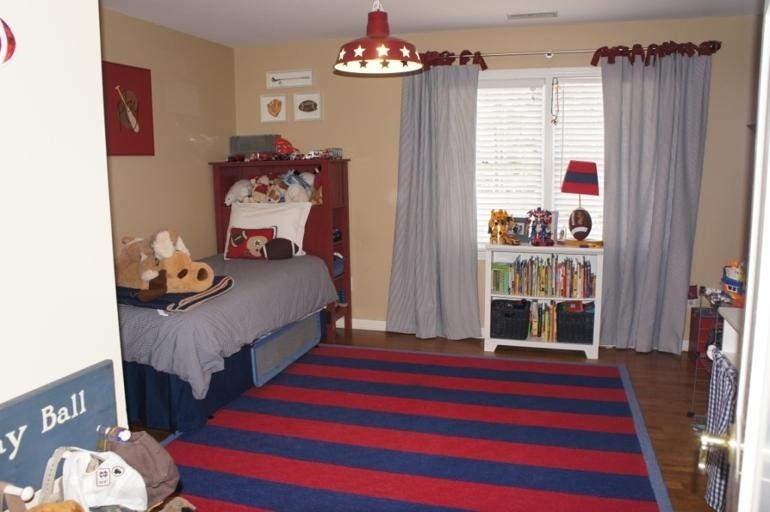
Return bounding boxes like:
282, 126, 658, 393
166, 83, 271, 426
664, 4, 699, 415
116, 252, 338, 433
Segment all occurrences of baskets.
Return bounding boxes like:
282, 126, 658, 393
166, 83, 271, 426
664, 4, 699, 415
556, 302, 594, 343
491, 300, 530, 339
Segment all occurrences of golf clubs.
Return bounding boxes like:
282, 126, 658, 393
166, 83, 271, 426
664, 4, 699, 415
272, 77, 310, 81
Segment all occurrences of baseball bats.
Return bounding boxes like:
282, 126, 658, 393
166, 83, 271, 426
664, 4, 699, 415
116, 85, 139, 133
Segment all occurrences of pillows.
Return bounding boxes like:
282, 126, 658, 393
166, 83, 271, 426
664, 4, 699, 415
223, 201, 313, 261
225, 226, 277, 259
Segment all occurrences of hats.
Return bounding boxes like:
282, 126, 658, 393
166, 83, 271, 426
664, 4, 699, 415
41, 446, 148, 512
91, 426, 180, 511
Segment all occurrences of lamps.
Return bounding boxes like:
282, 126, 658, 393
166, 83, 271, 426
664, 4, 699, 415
561, 160, 600, 245
332, 0, 424, 77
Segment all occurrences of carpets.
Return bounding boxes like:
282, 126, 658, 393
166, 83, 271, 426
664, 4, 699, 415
156, 341, 673, 512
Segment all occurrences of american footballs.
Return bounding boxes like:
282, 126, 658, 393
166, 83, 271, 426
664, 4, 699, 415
231, 230, 248, 247
260, 237, 298, 261
569, 209, 592, 240
298, 100, 317, 112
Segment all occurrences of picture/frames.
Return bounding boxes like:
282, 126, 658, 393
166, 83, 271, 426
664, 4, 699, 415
103, 59, 155, 156
293, 91, 323, 121
510, 217, 529, 242
259, 93, 288, 123
265, 70, 313, 90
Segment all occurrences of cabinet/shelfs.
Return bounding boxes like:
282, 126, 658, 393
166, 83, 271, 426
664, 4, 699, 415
484, 243, 605, 360
687, 286, 746, 432
208, 159, 353, 342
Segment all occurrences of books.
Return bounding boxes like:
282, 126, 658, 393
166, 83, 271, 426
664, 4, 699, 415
490, 253, 597, 344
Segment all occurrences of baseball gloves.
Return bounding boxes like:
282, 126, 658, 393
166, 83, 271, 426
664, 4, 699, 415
267, 99, 281, 116
117, 90, 138, 132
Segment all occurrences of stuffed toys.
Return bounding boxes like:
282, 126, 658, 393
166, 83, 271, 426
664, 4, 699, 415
223, 167, 323, 206
113, 228, 214, 303
